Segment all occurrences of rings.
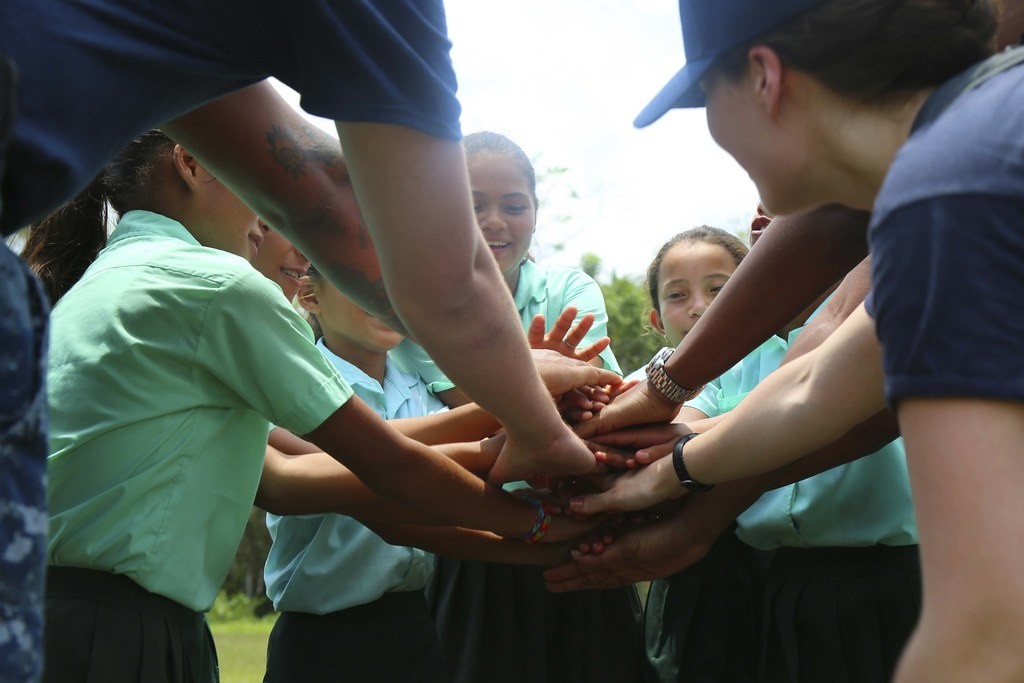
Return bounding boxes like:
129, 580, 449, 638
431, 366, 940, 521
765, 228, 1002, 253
564, 337, 577, 349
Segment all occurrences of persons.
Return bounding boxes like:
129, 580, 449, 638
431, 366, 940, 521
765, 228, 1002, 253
0, 0, 1024, 683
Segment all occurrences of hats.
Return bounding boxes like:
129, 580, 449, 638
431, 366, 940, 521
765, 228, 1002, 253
634, 0, 812, 129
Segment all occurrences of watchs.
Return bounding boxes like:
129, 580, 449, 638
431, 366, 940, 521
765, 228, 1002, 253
646, 346, 706, 403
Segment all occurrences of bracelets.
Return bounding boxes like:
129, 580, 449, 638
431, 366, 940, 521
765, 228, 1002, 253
509, 490, 545, 540
671, 433, 718, 494
527, 492, 552, 544
